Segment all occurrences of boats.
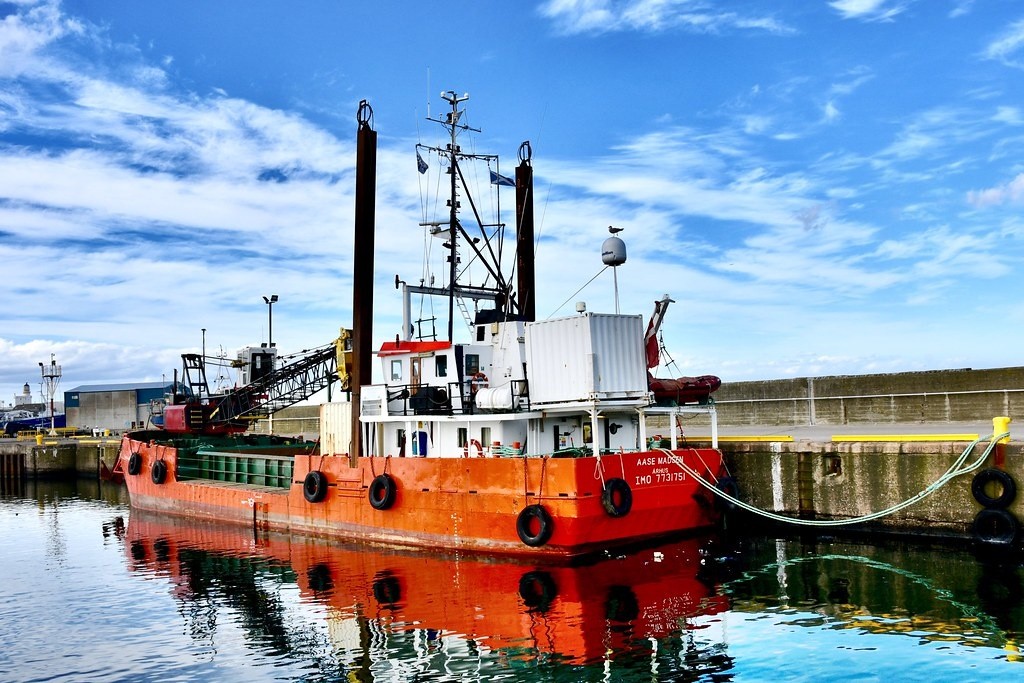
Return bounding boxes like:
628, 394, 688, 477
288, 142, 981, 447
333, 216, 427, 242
109, 87, 736, 566
639, 293, 723, 406
107, 499, 735, 683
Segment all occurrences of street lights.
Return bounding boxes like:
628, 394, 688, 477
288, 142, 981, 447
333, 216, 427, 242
262, 294, 278, 347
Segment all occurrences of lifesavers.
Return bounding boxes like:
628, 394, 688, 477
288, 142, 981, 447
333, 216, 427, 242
971, 466, 1020, 547
130, 539, 146, 560
517, 571, 558, 606
600, 478, 632, 518
306, 564, 334, 591
151, 460, 167, 484
128, 452, 141, 475
516, 504, 553, 547
153, 537, 170, 562
604, 585, 640, 621
714, 476, 740, 511
372, 569, 401, 604
303, 470, 328, 503
463, 438, 483, 458
472, 373, 489, 393
368, 473, 396, 510
